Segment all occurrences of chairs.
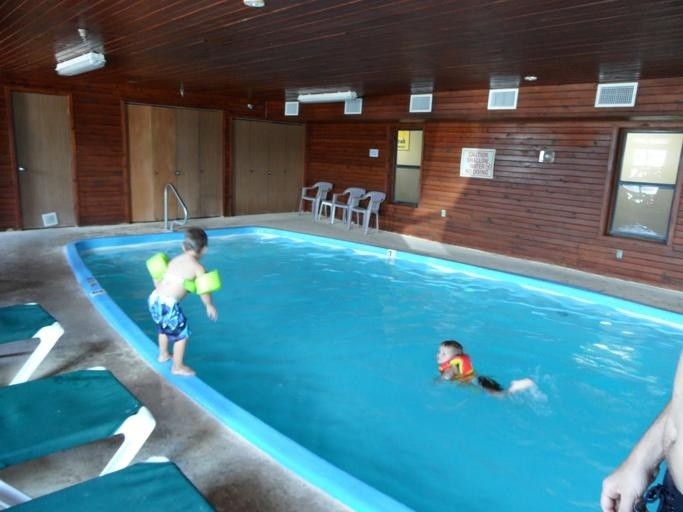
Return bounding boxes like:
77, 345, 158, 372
298, 181, 385, 235
1, 364, 159, 507
1, 453, 216, 512
0, 301, 65, 382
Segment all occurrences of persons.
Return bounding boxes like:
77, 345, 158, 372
597, 346, 682, 512
144, 225, 220, 377
432, 339, 549, 404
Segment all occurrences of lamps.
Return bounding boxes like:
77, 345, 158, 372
53, 47, 105, 77
293, 88, 359, 106
